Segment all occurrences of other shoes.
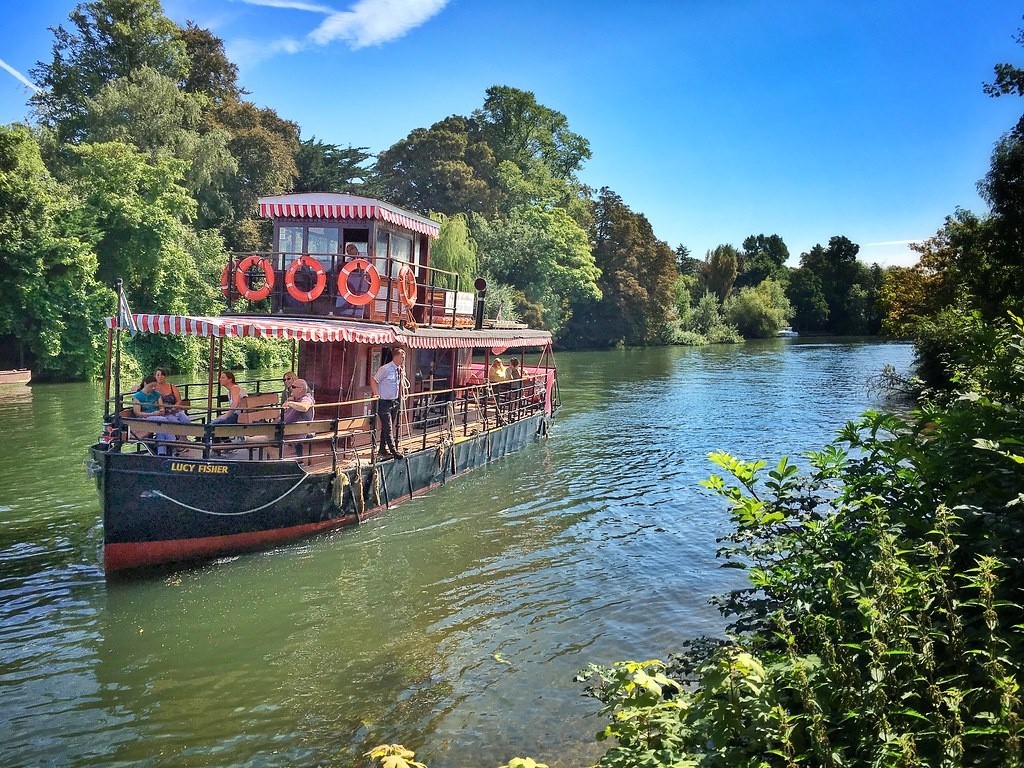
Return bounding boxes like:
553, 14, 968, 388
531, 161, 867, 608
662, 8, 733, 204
175, 447, 189, 455
208, 448, 221, 458
221, 449, 237, 454
378, 451, 391, 455
394, 452, 403, 459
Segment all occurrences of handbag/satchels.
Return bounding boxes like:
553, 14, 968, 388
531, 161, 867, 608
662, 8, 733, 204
284, 398, 314, 424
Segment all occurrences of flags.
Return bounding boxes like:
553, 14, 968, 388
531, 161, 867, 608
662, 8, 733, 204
122, 289, 137, 337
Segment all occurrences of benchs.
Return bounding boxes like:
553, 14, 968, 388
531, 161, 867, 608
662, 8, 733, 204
118, 398, 370, 466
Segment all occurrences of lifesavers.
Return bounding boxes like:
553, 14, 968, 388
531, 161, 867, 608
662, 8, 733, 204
285, 255, 327, 302
220, 258, 248, 300
236, 256, 275, 301
397, 265, 418, 307
338, 259, 382, 305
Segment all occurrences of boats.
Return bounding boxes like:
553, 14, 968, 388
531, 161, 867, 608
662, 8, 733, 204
87, 188, 560, 579
777, 326, 798, 337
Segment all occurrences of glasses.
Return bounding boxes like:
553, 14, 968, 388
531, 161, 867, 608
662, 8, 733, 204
291, 385, 302, 389
283, 377, 292, 381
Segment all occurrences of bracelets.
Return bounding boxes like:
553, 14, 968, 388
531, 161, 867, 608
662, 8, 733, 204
525, 373, 529, 375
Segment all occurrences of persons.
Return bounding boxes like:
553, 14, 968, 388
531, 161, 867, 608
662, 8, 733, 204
490, 357, 531, 416
132, 368, 191, 456
327, 243, 370, 274
371, 348, 406, 460
211, 371, 249, 454
237, 371, 315, 465
